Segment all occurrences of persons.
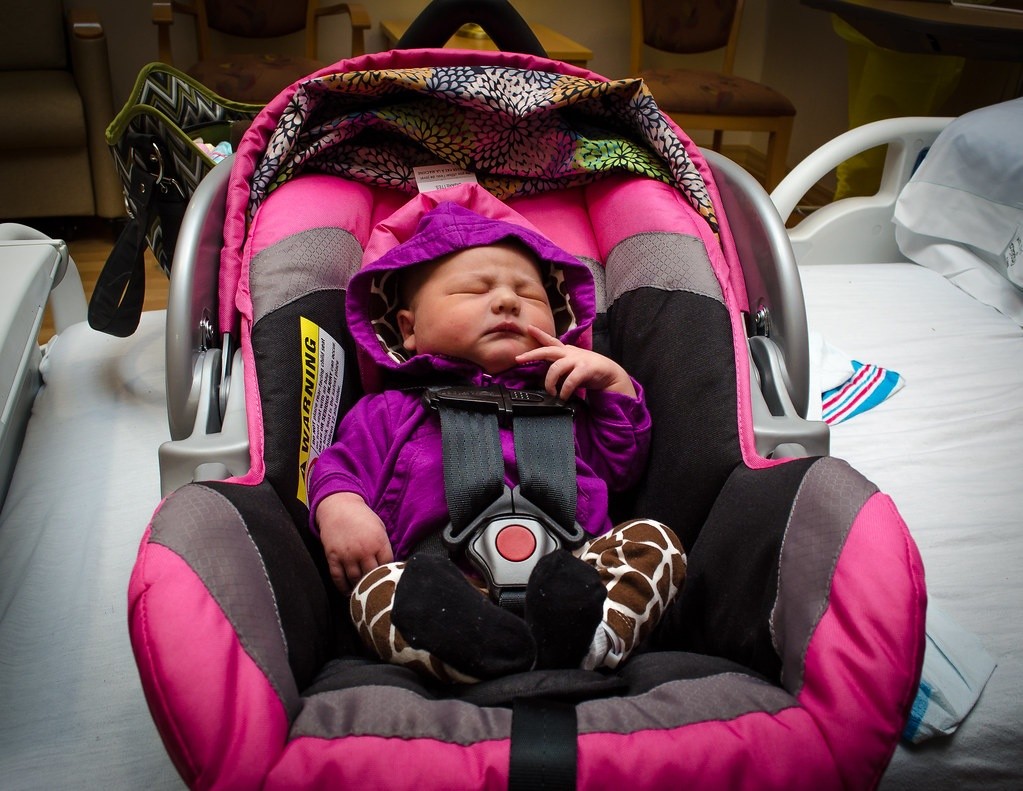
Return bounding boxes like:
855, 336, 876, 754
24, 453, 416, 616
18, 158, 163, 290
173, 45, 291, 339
306, 195, 689, 689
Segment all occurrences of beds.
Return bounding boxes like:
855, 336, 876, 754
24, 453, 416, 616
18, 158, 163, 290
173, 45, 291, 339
0, 114, 1023, 791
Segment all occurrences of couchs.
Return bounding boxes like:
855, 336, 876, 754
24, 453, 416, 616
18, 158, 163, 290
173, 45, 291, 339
0, 0, 129, 240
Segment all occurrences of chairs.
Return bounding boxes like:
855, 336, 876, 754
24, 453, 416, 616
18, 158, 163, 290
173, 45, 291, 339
151, 0, 370, 102
628, 0, 796, 195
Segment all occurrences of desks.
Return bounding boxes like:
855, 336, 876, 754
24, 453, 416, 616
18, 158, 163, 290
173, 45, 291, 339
380, 18, 593, 69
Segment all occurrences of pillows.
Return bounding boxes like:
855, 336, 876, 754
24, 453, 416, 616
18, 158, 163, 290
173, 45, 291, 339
890, 96, 1023, 326
